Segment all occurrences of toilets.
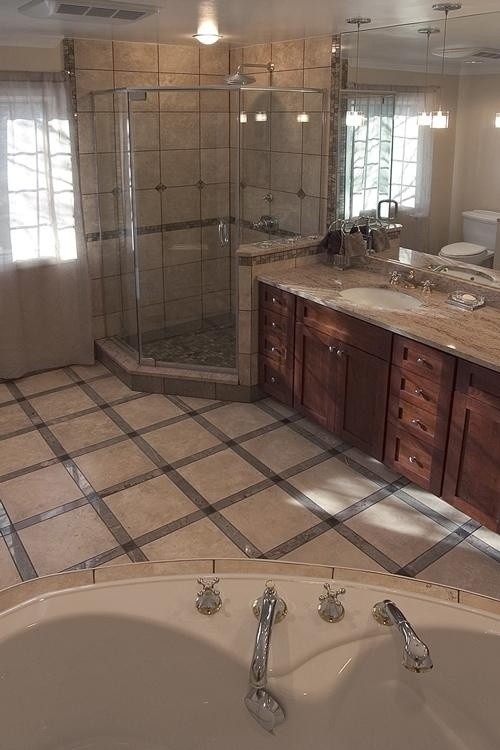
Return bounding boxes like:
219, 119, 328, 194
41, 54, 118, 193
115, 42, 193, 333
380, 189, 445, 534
438, 209, 500, 268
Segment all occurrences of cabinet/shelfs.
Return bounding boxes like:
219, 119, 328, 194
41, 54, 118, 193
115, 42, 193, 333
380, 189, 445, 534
383, 328, 457, 498
294, 294, 393, 462
440, 356, 500, 535
258, 280, 296, 407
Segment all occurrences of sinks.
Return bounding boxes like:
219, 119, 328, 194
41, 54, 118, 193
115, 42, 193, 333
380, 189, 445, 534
431, 265, 494, 284
0, 571, 500, 750
338, 285, 424, 309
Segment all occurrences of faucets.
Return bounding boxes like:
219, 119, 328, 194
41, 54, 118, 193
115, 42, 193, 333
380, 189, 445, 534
399, 270, 417, 290
432, 264, 448, 273
371, 597, 436, 675
248, 580, 288, 690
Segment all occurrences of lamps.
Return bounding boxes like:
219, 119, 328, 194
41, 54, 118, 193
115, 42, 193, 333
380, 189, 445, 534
418, 28, 440, 126
192, 33, 223, 46
433, 1, 460, 129
494, 113, 500, 129
238, 111, 309, 124
345, 17, 371, 125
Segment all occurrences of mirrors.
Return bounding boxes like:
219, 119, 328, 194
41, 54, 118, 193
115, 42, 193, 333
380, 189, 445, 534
326, 10, 500, 310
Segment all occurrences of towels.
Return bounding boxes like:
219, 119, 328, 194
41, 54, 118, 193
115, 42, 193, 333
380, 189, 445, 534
350, 225, 369, 240
367, 227, 391, 253
319, 230, 346, 255
345, 231, 366, 258
